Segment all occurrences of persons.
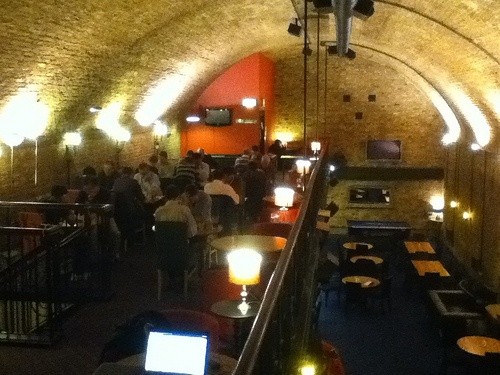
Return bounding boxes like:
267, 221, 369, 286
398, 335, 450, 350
154, 184, 204, 290
268, 140, 287, 168
40, 146, 286, 260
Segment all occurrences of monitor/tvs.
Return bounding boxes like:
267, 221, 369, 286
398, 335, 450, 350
366, 140, 402, 160
146, 329, 209, 375
205, 108, 230, 125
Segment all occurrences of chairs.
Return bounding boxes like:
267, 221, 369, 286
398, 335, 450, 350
253, 221, 292, 238
100, 308, 219, 363
412, 251, 439, 258
153, 221, 202, 300
356, 259, 376, 277
212, 194, 235, 238
356, 245, 368, 255
423, 272, 440, 289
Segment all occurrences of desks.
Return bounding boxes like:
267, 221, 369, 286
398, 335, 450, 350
280, 155, 303, 183
115, 351, 237, 375
347, 220, 416, 239
210, 236, 287, 253
262, 193, 303, 203
197, 222, 223, 236
404, 241, 437, 255
456, 336, 500, 356
411, 260, 451, 277
342, 242, 383, 288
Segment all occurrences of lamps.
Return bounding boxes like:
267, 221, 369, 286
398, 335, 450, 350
227, 249, 263, 309
295, 160, 311, 188
